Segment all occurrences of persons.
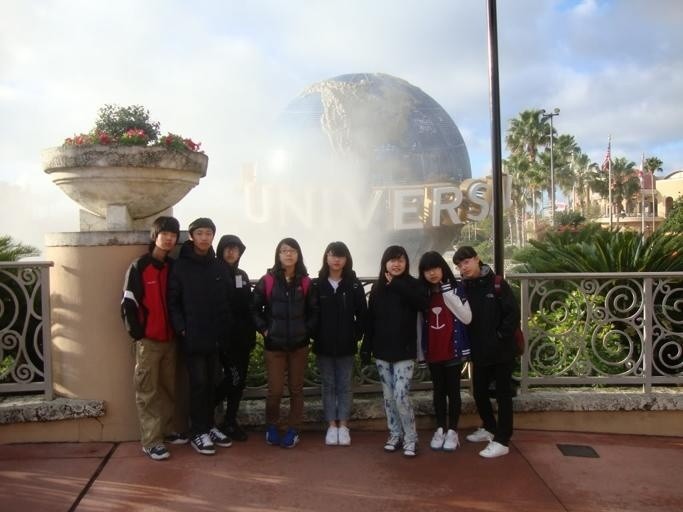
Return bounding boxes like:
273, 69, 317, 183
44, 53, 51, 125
452, 246, 524, 459
310, 240, 368, 447
247, 237, 319, 448
415, 251, 472, 451
359, 246, 426, 458
166, 217, 232, 455
209, 235, 256, 442
121, 217, 188, 460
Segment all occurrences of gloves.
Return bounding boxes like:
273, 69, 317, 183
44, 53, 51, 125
359, 351, 372, 375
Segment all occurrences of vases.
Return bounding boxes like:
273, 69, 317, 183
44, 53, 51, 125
40, 146, 207, 218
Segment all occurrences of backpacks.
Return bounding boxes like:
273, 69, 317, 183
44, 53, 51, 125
493, 274, 525, 353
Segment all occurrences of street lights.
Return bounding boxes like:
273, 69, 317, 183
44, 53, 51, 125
543, 113, 560, 224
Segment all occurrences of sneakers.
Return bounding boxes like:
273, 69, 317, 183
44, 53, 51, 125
220, 421, 248, 441
165, 431, 189, 445
190, 432, 217, 455
324, 425, 351, 446
466, 427, 495, 441
383, 435, 402, 451
142, 441, 170, 461
265, 427, 280, 445
403, 440, 419, 457
479, 441, 510, 458
430, 426, 459, 451
280, 432, 305, 448
209, 427, 232, 448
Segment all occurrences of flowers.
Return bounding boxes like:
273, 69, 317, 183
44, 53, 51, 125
60, 102, 203, 148
